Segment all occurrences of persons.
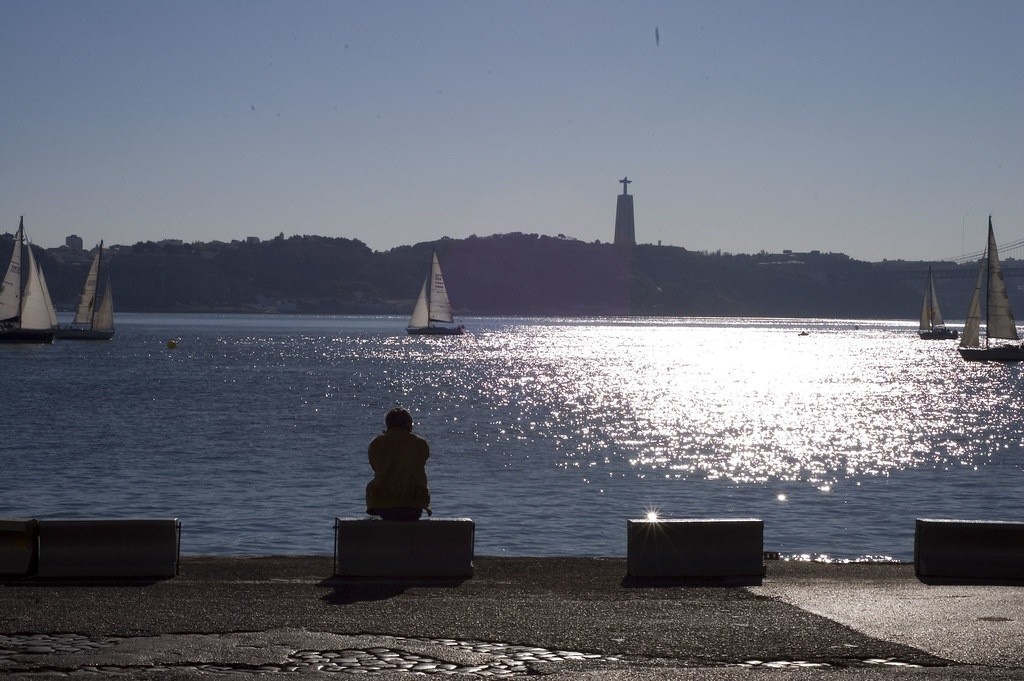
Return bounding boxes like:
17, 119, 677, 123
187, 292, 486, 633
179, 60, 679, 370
365, 407, 432, 519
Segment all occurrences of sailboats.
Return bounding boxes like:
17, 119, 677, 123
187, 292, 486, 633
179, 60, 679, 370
916, 263, 958, 340
404, 244, 465, 336
956, 212, 1024, 362
0, 215, 59, 344
55, 237, 117, 340
